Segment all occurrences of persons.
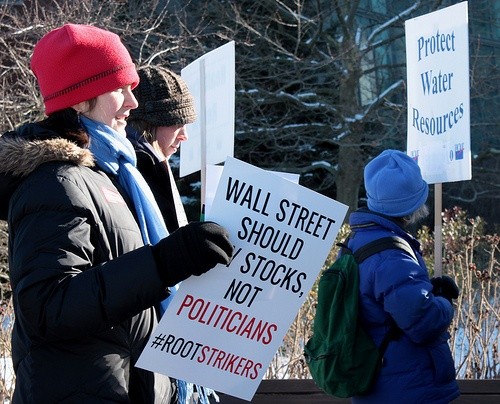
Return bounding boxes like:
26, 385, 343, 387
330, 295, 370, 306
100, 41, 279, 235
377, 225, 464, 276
125, 65, 198, 234
0, 23, 235, 404
336, 149, 460, 404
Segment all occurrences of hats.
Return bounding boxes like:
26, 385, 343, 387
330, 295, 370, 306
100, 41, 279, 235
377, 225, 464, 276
129, 65, 196, 126
363, 149, 429, 216
31, 24, 140, 116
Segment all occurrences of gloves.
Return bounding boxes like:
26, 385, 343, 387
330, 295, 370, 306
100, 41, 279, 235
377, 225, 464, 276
149, 221, 235, 288
430, 276, 459, 307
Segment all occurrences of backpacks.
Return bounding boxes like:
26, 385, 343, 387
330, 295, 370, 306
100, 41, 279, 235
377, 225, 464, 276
303, 232, 419, 398
